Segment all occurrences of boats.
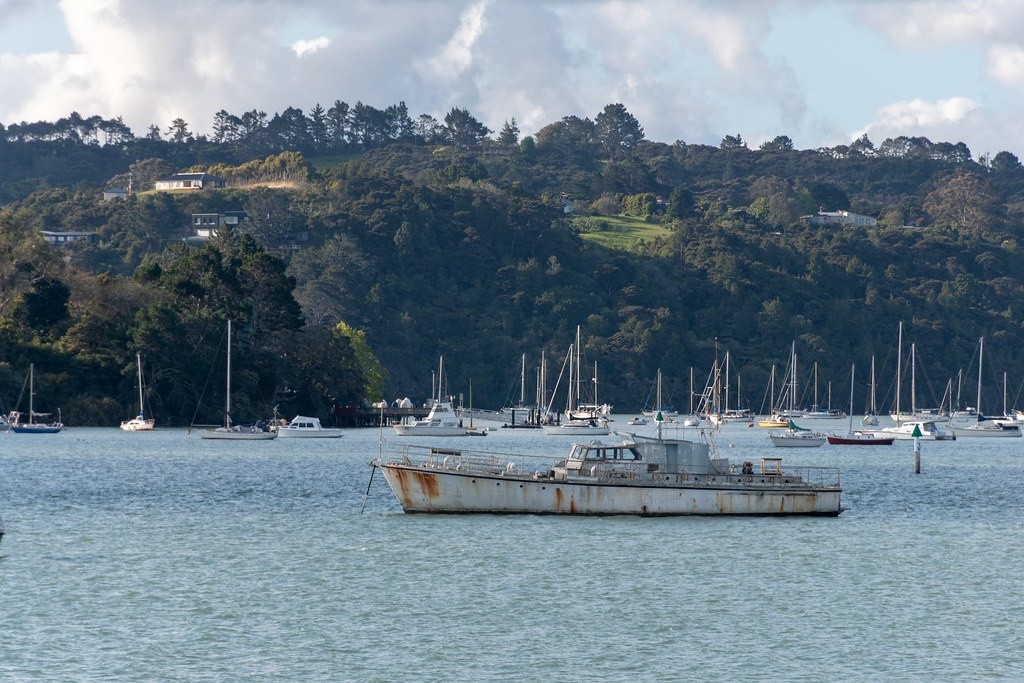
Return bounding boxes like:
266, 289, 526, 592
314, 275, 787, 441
391, 354, 468, 436
267, 415, 344, 438
367, 410, 852, 516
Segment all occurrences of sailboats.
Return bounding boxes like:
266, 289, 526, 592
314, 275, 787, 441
768, 338, 826, 447
120, 353, 156, 431
943, 337, 1023, 437
496, 324, 615, 435
625, 331, 1024, 427
0, 362, 64, 433
872, 321, 956, 440
827, 363, 895, 445
184, 318, 279, 440
